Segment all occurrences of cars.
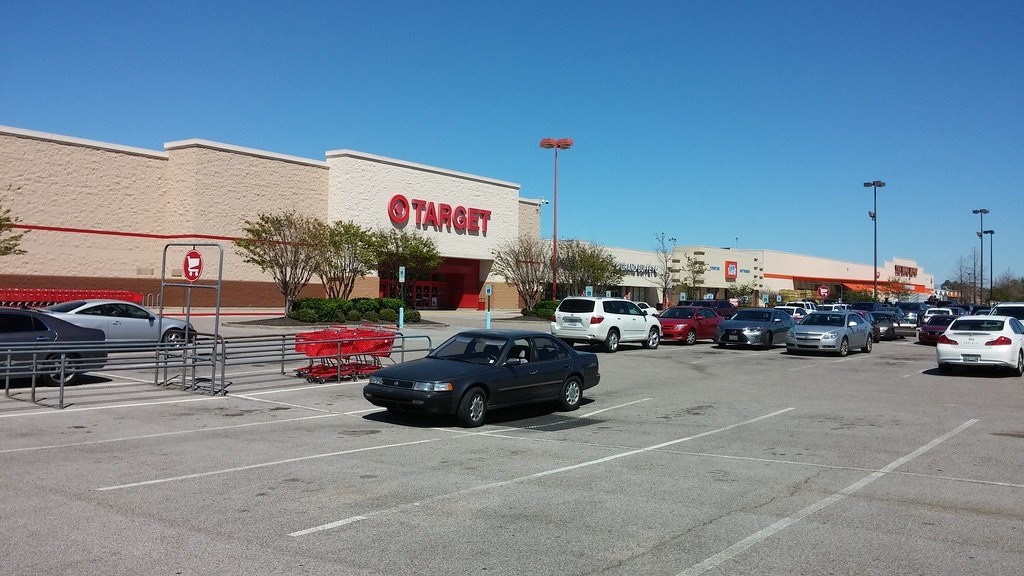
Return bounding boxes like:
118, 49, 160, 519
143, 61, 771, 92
605, 301, 660, 315
827, 310, 880, 343
363, 329, 601, 427
655, 306, 725, 345
762, 306, 809, 323
29, 299, 197, 351
817, 304, 843, 311
922, 308, 953, 323
919, 315, 958, 344
937, 301, 1024, 328
936, 316, 1024, 377
850, 302, 928, 341
0, 307, 107, 387
716, 308, 797, 350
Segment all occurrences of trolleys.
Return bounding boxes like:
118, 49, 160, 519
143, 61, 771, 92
293, 324, 399, 384
0, 288, 143, 308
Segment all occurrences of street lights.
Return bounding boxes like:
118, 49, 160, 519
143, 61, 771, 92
983, 230, 995, 300
863, 181, 886, 300
972, 209, 989, 306
540, 138, 573, 301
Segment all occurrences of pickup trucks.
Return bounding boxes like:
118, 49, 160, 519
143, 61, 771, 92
784, 311, 874, 357
785, 302, 816, 315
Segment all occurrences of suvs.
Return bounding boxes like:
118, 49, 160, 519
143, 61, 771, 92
550, 296, 661, 353
688, 299, 737, 316
